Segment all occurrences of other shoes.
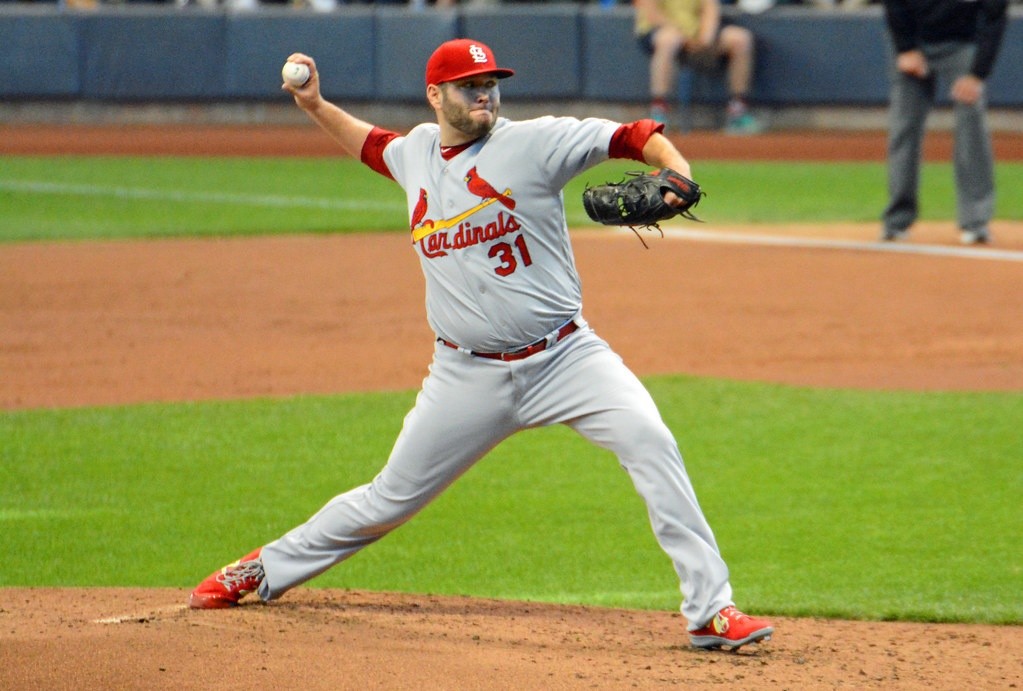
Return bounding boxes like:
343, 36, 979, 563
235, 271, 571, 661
962, 225, 986, 247
884, 223, 906, 245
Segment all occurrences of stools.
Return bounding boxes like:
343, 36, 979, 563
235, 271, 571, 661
677, 52, 732, 131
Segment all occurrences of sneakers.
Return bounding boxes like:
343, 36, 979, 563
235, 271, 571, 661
189, 547, 262, 607
685, 606, 777, 646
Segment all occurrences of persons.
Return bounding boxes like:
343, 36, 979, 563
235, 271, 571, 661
189, 38, 774, 651
878, 1, 1011, 247
632, 1, 771, 137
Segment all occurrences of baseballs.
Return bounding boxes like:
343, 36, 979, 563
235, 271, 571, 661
283, 61, 311, 88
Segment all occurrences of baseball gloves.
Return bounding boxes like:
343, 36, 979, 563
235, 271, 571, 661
582, 165, 702, 229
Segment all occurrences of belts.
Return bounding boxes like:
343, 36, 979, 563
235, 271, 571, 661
436, 318, 576, 361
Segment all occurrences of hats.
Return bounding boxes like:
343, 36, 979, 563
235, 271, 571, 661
425, 39, 514, 88
